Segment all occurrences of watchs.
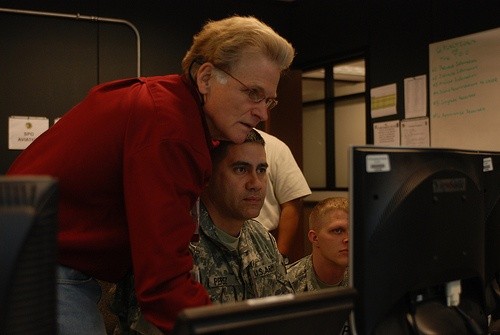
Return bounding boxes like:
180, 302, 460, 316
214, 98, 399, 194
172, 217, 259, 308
281, 253, 290, 265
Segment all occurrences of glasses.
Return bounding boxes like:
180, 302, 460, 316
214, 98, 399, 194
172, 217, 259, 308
213, 63, 279, 110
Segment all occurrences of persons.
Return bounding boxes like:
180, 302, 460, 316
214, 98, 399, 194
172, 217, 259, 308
252, 126, 312, 265
107, 129, 295, 335
285, 197, 350, 335
6, 16, 295, 335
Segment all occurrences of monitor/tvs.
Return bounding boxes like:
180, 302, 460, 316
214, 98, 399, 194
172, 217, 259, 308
348, 144, 500, 335
172, 287, 356, 335
0, 175, 59, 335
